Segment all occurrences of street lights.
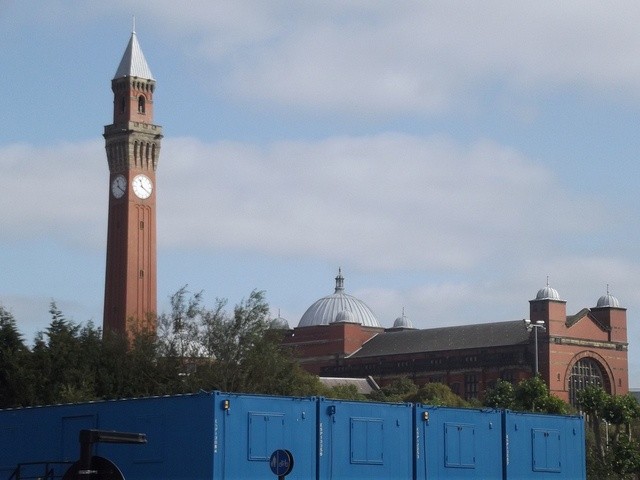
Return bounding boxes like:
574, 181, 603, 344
523, 319, 546, 373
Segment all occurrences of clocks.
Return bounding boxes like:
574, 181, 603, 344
111, 174, 127, 200
132, 174, 153, 200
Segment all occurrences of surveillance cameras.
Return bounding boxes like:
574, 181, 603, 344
522, 319, 531, 324
536, 320, 545, 325
